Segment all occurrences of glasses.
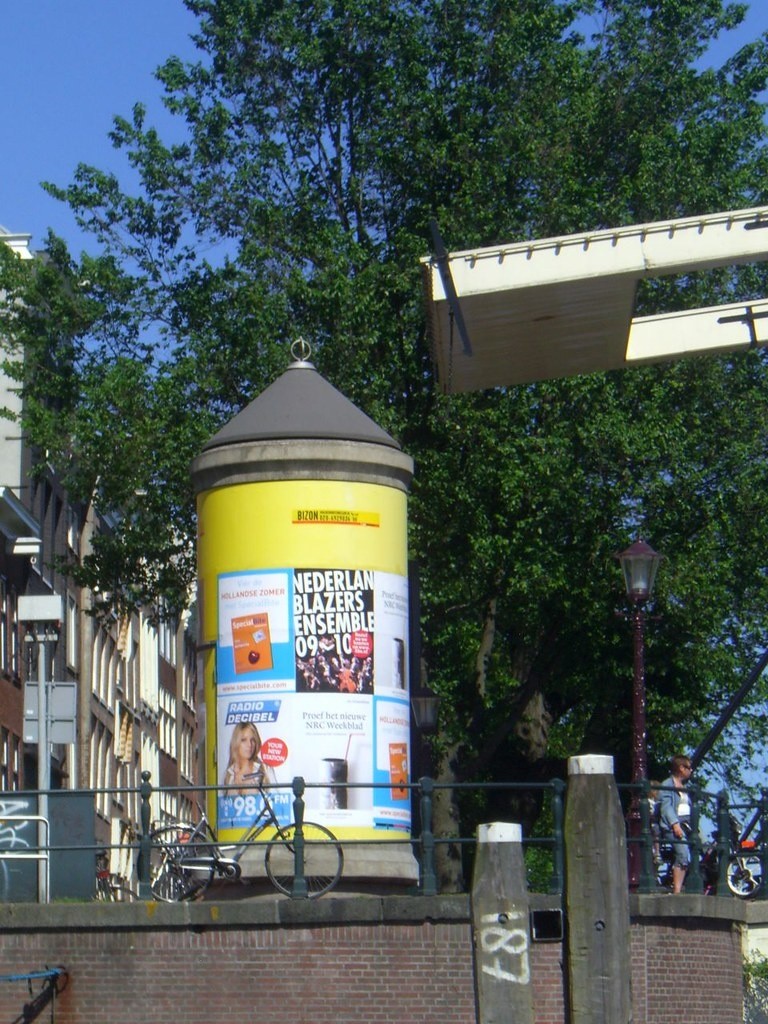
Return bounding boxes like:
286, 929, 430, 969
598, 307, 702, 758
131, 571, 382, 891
683, 765, 692, 770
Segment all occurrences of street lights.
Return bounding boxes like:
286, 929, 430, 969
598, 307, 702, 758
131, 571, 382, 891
617, 530, 659, 887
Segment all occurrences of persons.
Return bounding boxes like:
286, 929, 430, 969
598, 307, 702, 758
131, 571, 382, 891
223, 721, 270, 796
302, 637, 373, 691
647, 755, 693, 894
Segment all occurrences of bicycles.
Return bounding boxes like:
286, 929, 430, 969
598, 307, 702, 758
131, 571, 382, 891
95, 841, 115, 900
134, 769, 343, 901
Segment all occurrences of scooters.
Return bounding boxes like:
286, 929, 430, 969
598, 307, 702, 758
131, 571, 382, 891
653, 811, 766, 899
